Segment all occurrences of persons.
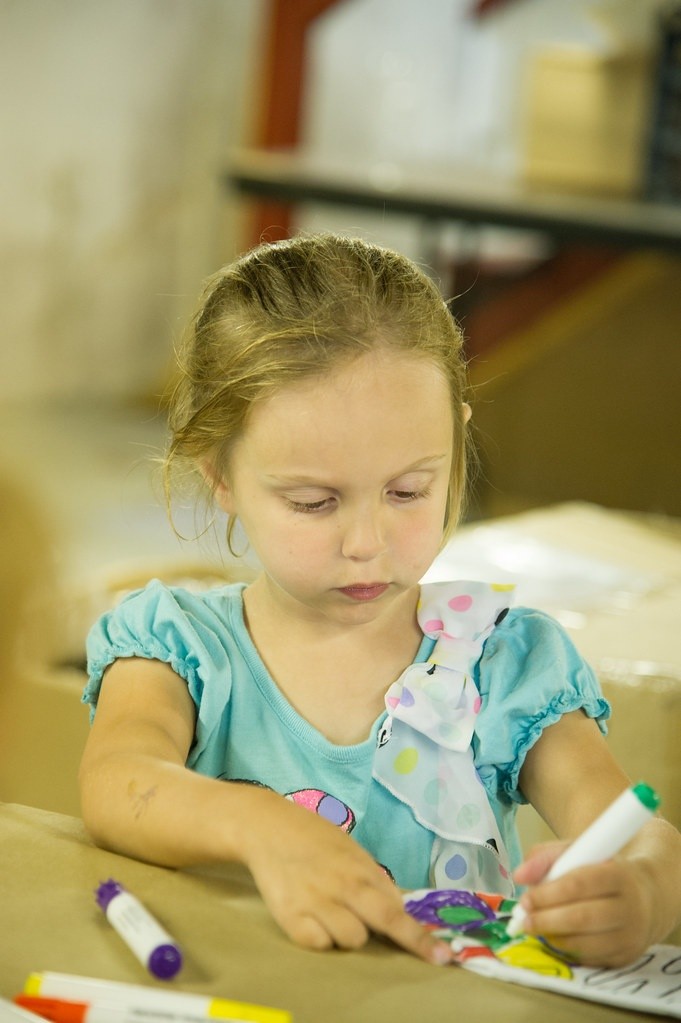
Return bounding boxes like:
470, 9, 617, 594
76, 236, 681, 969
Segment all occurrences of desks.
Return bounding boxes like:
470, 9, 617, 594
0, 804, 681, 1023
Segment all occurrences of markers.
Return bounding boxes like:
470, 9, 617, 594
9, 970, 290, 1023
93, 879, 183, 977
501, 779, 665, 942
